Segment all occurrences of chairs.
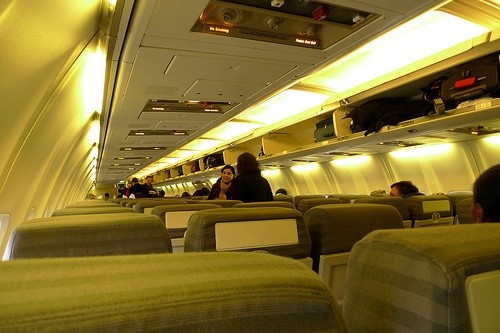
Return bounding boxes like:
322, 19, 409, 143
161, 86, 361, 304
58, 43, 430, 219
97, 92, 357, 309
0, 192, 500, 333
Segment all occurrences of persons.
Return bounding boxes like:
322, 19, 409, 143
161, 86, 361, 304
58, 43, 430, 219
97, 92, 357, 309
225, 152, 275, 202
205, 164, 236, 200
103, 176, 210, 199
471, 163, 500, 224
389, 181, 419, 198
275, 188, 288, 197
